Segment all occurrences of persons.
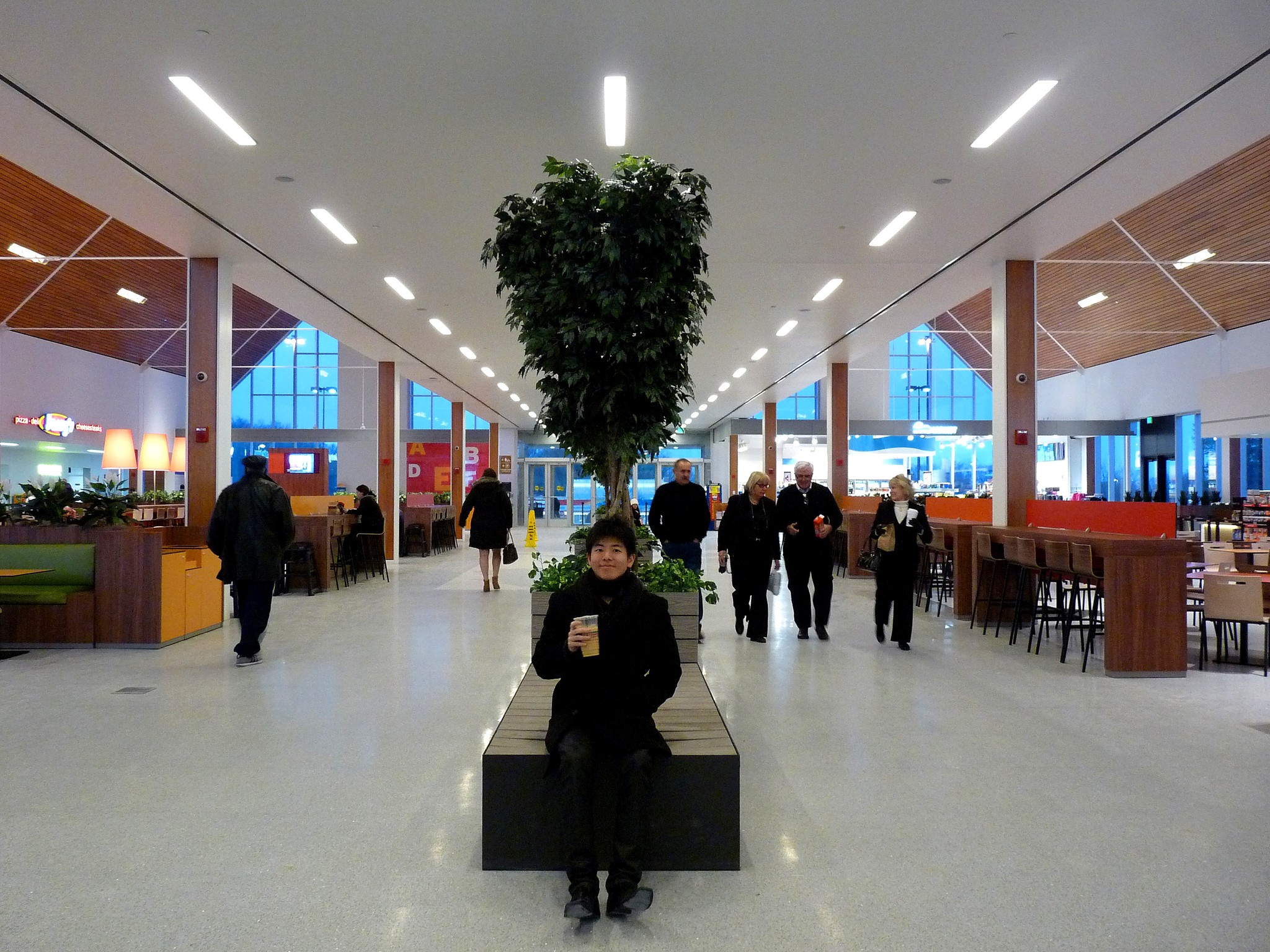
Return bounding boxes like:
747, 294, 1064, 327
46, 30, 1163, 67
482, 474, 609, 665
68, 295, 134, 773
459, 468, 513, 592
776, 461, 843, 640
63, 479, 74, 495
718, 472, 780, 643
343, 484, 384, 563
648, 458, 711, 639
554, 495, 561, 519
179, 484, 185, 493
630, 498, 643, 527
532, 517, 682, 920
870, 474, 934, 651
63, 504, 76, 521
207, 456, 296, 667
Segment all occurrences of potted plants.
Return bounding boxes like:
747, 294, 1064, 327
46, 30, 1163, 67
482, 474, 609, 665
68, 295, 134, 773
1178, 490, 1221, 519
485, 149, 713, 664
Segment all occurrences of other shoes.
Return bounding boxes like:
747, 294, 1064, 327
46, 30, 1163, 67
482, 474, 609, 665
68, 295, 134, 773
750, 636, 766, 643
876, 624, 884, 643
898, 641, 910, 651
815, 625, 829, 641
235, 655, 264, 667
563, 882, 601, 922
735, 615, 745, 635
797, 627, 809, 640
605, 881, 653, 919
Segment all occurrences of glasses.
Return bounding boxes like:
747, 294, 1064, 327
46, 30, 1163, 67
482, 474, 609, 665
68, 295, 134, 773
756, 483, 770, 488
795, 474, 813, 480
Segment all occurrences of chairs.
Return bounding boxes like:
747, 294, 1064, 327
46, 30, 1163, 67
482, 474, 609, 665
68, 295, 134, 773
1252, 542, 1270, 551
1198, 574, 1270, 677
1203, 542, 1239, 573
133, 507, 185, 528
1259, 537, 1270, 543
1253, 553, 1270, 574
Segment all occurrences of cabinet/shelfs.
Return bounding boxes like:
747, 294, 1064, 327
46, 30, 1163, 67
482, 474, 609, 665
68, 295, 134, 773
160, 543, 223, 649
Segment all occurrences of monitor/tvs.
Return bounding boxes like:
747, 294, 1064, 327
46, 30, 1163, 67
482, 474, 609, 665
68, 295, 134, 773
287, 454, 315, 473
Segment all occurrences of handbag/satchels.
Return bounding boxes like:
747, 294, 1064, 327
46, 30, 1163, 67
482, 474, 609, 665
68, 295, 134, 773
502, 529, 519, 564
877, 523, 896, 552
856, 535, 882, 573
767, 568, 781, 596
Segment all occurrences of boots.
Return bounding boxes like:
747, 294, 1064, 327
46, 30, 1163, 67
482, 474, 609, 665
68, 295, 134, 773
492, 576, 500, 590
483, 579, 490, 592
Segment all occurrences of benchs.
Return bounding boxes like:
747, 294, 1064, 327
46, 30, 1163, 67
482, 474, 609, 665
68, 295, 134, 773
480, 665, 741, 870
0, 544, 96, 649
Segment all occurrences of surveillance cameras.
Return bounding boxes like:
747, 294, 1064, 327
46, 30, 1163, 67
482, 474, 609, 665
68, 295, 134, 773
196, 371, 208, 382
1015, 373, 1027, 384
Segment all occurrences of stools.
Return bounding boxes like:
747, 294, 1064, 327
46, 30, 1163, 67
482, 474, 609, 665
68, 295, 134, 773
279, 518, 390, 596
835, 513, 848, 578
914, 526, 1104, 672
403, 504, 458, 558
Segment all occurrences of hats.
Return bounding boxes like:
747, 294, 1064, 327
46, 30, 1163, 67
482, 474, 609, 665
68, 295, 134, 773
241, 455, 267, 469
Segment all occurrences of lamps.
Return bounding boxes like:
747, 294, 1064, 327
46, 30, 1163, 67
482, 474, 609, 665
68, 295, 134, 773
138, 433, 170, 505
101, 428, 138, 498
170, 437, 186, 506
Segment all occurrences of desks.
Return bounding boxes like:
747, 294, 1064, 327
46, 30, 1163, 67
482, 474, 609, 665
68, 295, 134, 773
1183, 541, 1270, 668
971, 526, 1188, 679
404, 505, 451, 557
290, 514, 361, 592
848, 510, 992, 620
0, 569, 55, 661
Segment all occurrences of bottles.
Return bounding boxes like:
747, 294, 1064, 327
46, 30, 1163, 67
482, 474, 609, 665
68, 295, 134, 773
719, 553, 727, 573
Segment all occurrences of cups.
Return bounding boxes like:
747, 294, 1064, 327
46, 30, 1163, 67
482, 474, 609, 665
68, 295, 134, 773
574, 614, 600, 656
906, 509, 918, 527
